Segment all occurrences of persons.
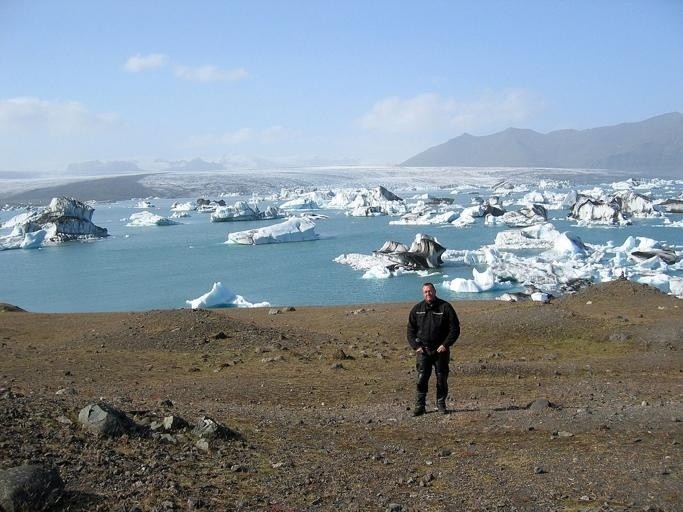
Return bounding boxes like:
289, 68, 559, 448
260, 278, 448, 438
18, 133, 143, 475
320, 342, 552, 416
406, 282, 460, 417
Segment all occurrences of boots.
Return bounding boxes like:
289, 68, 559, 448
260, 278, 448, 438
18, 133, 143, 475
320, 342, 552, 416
437, 397, 445, 414
413, 391, 426, 415
